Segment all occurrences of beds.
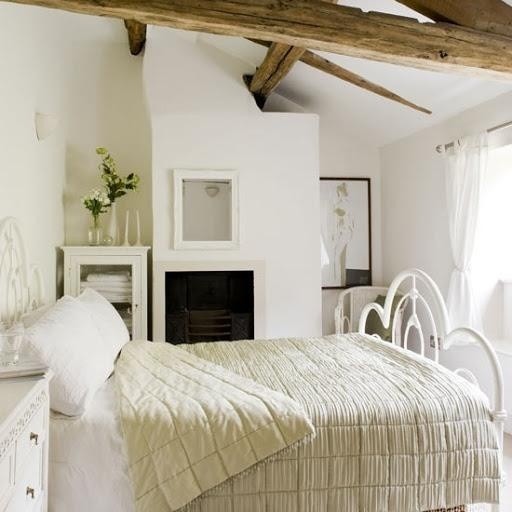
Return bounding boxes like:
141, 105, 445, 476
0, 217, 508, 512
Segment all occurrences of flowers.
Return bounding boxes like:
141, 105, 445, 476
95, 145, 141, 201
80, 188, 108, 226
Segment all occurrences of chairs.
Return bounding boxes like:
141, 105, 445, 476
333, 281, 412, 348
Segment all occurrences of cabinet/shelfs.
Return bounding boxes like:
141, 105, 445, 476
0, 365, 55, 512
59, 244, 152, 342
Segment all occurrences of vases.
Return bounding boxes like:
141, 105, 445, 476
88, 226, 102, 247
107, 202, 123, 246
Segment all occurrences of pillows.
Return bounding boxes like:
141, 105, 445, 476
367, 291, 402, 343
16, 286, 132, 419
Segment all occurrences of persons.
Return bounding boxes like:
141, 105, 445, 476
331, 179, 355, 283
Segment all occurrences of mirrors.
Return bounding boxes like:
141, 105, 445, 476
173, 169, 240, 251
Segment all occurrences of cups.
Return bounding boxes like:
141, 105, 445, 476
0, 322, 24, 368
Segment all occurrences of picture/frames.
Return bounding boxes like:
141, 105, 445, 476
319, 176, 373, 290
